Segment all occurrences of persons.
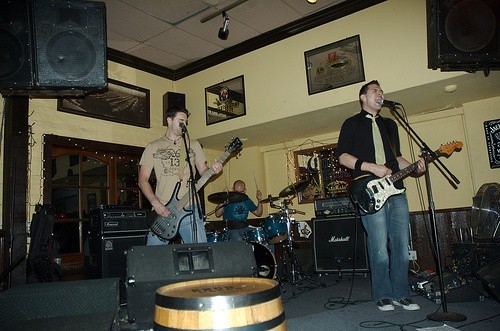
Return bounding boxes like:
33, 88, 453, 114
337, 79, 427, 311
137, 104, 223, 245
215, 180, 263, 220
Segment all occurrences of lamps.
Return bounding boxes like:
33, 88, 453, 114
218, 11, 230, 40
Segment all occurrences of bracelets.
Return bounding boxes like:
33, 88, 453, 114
355, 159, 364, 171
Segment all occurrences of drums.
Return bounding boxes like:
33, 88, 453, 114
246, 227, 275, 255
205, 232, 226, 242
260, 214, 290, 244
251, 241, 276, 279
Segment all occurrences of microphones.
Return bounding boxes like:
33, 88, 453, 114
380, 99, 401, 105
260, 267, 269, 271
179, 121, 187, 133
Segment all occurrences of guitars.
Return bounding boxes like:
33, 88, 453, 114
146, 136, 242, 240
348, 139, 464, 214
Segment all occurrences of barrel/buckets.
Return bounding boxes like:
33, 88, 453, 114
154, 277, 287, 330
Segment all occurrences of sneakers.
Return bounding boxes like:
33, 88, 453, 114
377, 299, 394, 311
392, 297, 420, 310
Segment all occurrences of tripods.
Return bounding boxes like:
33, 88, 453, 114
269, 200, 325, 297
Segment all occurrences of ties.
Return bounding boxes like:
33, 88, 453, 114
366, 114, 386, 165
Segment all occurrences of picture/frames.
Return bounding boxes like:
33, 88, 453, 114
57, 78, 150, 128
205, 75, 246, 126
304, 34, 365, 95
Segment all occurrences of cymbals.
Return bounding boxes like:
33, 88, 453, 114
208, 191, 248, 204
260, 197, 280, 203
279, 181, 306, 197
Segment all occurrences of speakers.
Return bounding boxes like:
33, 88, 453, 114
310, 215, 371, 274
119, 241, 258, 324
426, 0, 500, 77
162, 92, 186, 126
85, 235, 148, 279
0, 0, 109, 97
